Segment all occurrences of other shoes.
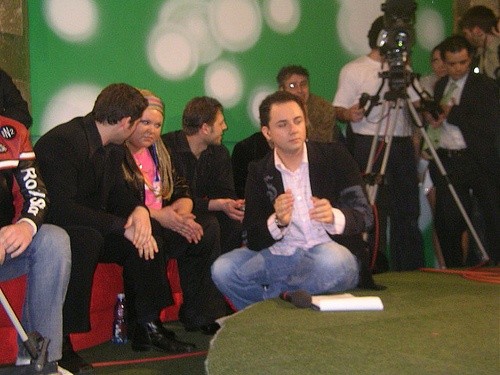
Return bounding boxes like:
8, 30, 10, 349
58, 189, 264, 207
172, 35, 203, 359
25, 360, 73, 375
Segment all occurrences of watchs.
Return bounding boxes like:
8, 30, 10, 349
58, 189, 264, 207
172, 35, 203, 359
275, 216, 289, 229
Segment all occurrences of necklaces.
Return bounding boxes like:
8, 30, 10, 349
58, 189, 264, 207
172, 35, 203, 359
133, 149, 143, 169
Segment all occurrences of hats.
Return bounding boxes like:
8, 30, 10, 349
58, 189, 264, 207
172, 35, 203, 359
138, 89, 163, 114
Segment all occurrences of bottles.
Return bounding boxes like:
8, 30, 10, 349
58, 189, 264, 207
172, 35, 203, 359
111, 293, 128, 344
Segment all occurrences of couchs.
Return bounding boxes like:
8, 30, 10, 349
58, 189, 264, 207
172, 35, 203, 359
0, 254, 233, 368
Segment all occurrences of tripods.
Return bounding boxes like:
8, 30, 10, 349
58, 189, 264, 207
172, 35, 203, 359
359, 68, 488, 260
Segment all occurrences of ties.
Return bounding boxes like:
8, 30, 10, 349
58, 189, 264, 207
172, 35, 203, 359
421, 81, 456, 151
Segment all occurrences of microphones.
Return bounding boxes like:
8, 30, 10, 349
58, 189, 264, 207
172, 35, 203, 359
280, 290, 312, 308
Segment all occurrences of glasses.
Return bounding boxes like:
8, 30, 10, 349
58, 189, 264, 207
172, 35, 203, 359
284, 80, 308, 89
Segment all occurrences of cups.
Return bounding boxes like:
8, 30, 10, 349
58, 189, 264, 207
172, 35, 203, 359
239, 205, 245, 212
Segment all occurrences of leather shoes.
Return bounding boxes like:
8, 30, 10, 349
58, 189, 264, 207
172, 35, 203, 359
131, 329, 196, 354
147, 314, 175, 338
59, 348, 93, 375
209, 299, 235, 319
180, 311, 223, 337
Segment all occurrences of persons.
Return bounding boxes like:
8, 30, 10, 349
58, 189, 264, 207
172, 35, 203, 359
110, 89, 221, 338
413, 33, 500, 271
278, 65, 345, 142
456, 6, 500, 77
0, 67, 33, 129
333, 14, 423, 269
162, 97, 246, 323
212, 92, 374, 311
0, 114, 71, 375
32, 84, 197, 375
